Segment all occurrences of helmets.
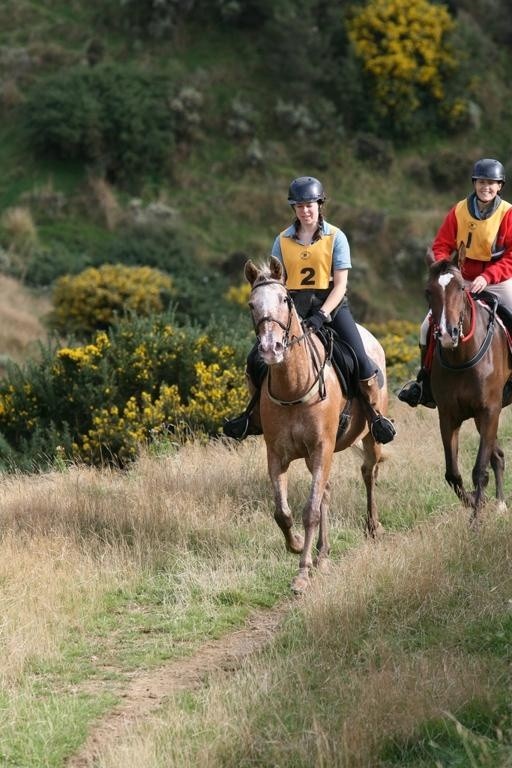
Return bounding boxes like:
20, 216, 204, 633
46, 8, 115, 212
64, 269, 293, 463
287, 177, 326, 204
472, 158, 506, 183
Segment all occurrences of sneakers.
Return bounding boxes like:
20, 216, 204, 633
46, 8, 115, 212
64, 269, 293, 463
401, 384, 437, 409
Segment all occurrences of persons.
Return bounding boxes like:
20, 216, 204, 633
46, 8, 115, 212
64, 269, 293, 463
394, 157, 512, 410
222, 174, 397, 446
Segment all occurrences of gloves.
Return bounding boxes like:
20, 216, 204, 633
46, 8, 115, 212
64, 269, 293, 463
307, 310, 327, 333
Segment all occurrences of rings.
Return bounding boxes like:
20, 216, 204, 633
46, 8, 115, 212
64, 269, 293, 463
478, 286, 481, 289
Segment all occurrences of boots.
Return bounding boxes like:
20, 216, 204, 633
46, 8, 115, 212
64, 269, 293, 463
360, 374, 395, 444
223, 369, 263, 437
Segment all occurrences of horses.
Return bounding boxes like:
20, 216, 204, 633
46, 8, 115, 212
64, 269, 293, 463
243, 255, 395, 596
425, 240, 512, 532
